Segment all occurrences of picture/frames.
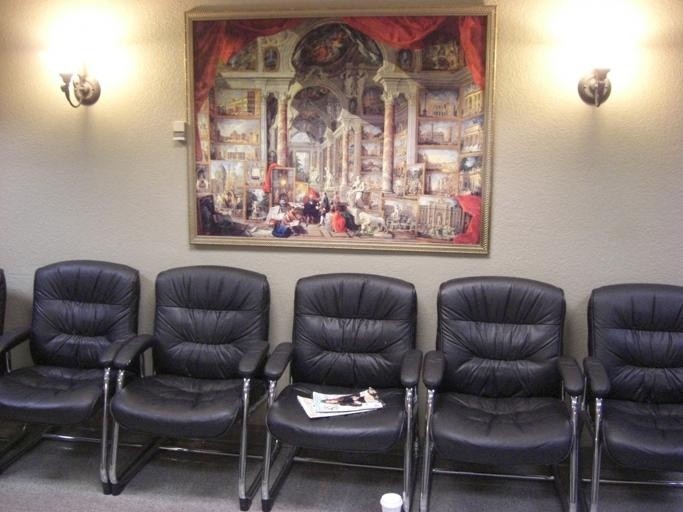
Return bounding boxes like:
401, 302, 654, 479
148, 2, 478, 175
181, 7, 497, 256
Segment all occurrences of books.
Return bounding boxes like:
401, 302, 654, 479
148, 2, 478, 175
296, 386, 386, 419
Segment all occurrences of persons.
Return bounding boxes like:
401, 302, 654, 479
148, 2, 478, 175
303, 191, 361, 229
265, 193, 303, 237
246, 183, 257, 220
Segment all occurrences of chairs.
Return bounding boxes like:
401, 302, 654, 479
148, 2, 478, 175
582, 283, 683, 511
421, 275, 585, 512
110, 266, 270, 510
260, 271, 422, 511
1, 260, 139, 496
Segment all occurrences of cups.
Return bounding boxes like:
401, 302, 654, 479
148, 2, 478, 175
379, 492, 403, 512
